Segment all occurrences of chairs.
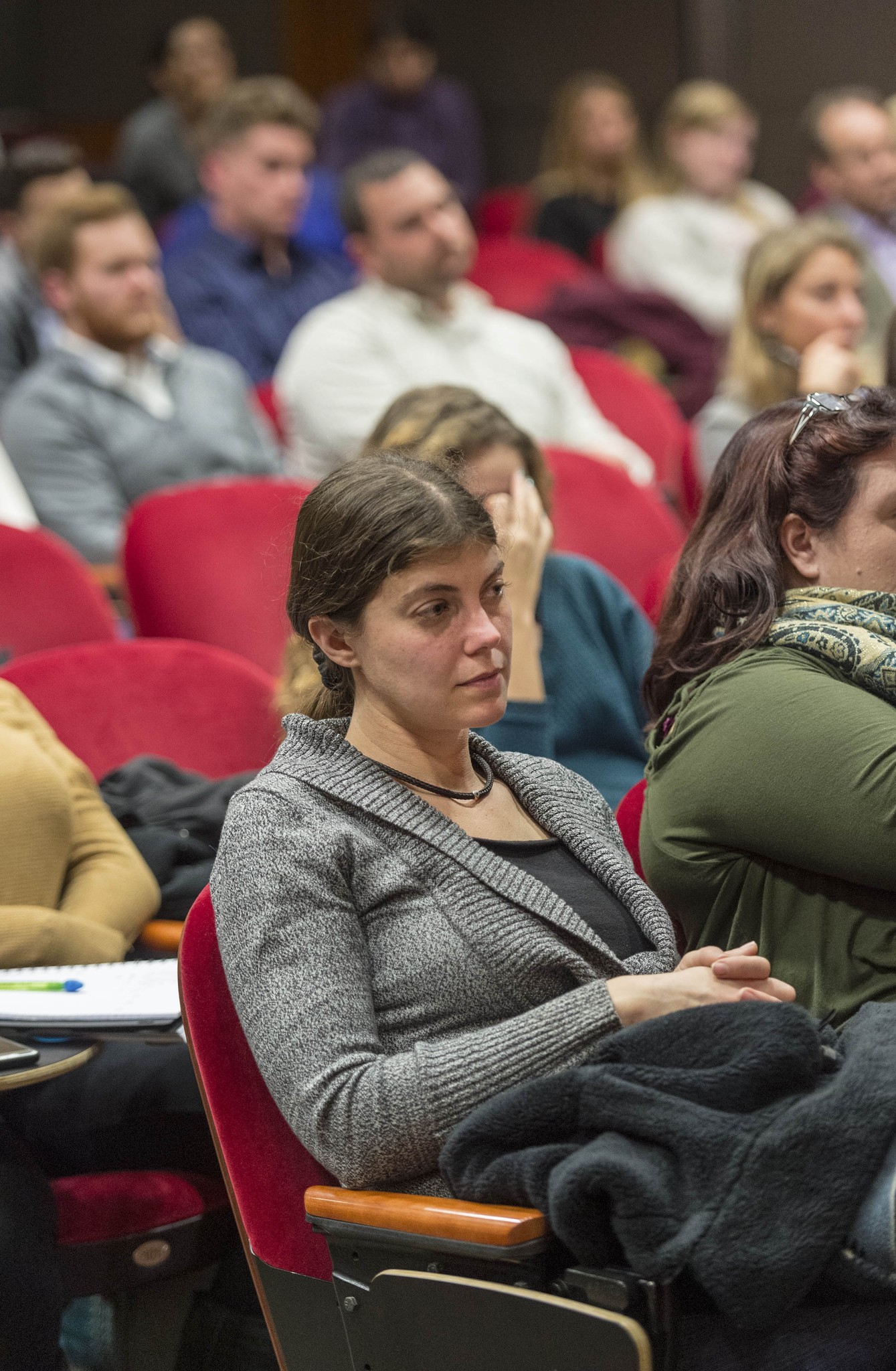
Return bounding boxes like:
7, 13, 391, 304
0, 184, 896, 1371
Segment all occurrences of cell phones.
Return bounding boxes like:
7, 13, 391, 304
0, 1035, 40, 1070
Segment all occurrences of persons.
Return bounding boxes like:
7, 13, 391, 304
159, 77, 357, 369
603, 79, 792, 334
319, 14, 492, 216
113, 14, 239, 191
277, 386, 663, 816
639, 383, 896, 1028
262, 145, 660, 502
523, 74, 668, 257
797, 88, 896, 296
205, 453, 896, 1369
1, 680, 266, 1370
689, 218, 889, 492
0, 125, 180, 388
3, 182, 299, 613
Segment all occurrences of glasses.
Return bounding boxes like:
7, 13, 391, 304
785, 385, 874, 457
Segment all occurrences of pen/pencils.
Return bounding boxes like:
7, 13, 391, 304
0, 980, 84, 992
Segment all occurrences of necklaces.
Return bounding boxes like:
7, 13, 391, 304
365, 744, 498, 804
